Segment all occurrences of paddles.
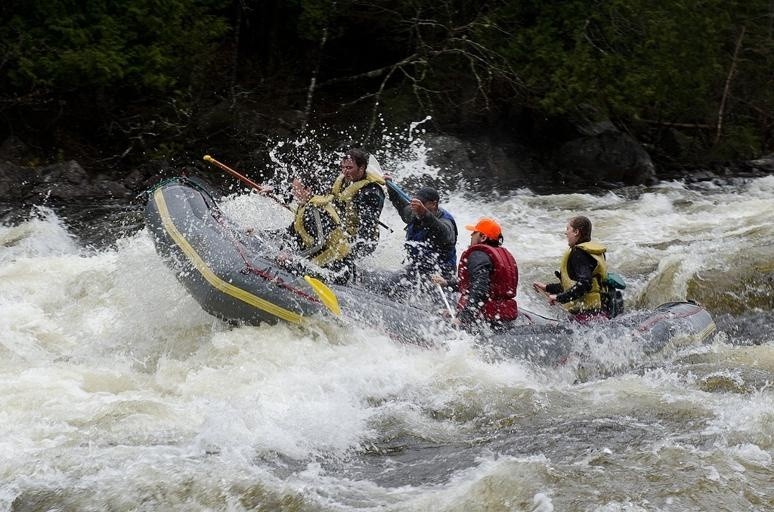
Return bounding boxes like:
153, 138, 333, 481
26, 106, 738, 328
253, 234, 339, 315
366, 154, 413, 204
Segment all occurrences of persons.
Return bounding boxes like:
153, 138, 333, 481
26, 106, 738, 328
381, 167, 459, 302
274, 173, 354, 287
430, 214, 520, 339
252, 145, 386, 287
532, 214, 625, 333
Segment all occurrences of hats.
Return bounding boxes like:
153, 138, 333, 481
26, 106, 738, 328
464, 218, 502, 243
412, 186, 440, 205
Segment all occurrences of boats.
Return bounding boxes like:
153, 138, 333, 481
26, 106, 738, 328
143, 172, 721, 384
